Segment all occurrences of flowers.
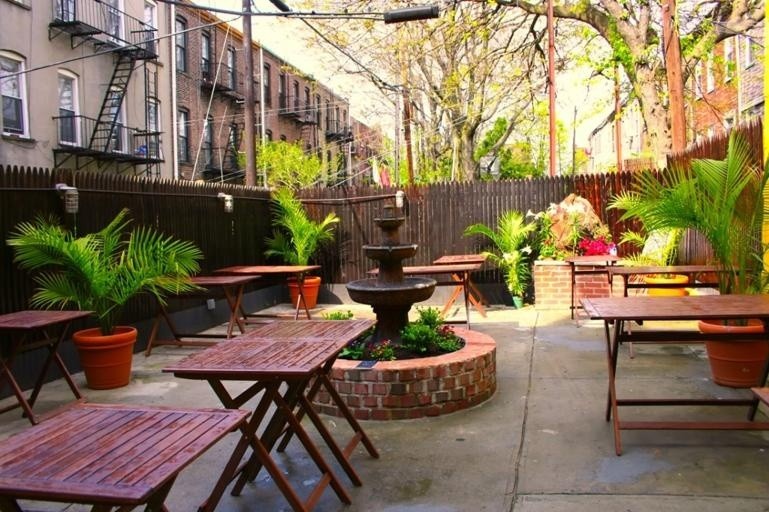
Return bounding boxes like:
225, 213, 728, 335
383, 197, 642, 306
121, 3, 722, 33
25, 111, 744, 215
578, 236, 617, 256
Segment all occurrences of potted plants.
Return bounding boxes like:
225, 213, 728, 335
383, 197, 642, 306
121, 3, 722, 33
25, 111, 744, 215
6, 201, 210, 390
457, 210, 540, 310
261, 180, 340, 311
606, 121, 768, 388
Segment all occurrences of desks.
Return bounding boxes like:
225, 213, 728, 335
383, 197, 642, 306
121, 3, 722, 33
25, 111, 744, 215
604, 265, 768, 358
0, 309, 97, 426
245, 316, 380, 488
563, 258, 619, 319
0, 397, 252, 511
576, 293, 769, 457
366, 262, 485, 329
432, 253, 488, 319
143, 275, 265, 357
215, 266, 320, 326
162, 335, 352, 510
